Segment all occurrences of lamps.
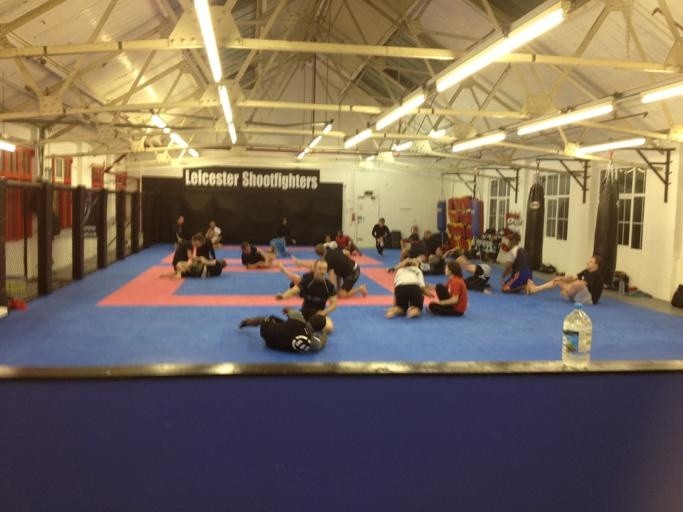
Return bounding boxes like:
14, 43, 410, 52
296, 122, 334, 159
517, 102, 614, 136
376, 90, 426, 132
452, 131, 506, 153
394, 141, 411, 152
436, 1, 571, 93
570, 136, 647, 158
192, 0, 242, 144
344, 128, 373, 149
430, 129, 445, 139
641, 81, 682, 104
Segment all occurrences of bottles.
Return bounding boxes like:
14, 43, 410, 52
560, 302, 593, 371
